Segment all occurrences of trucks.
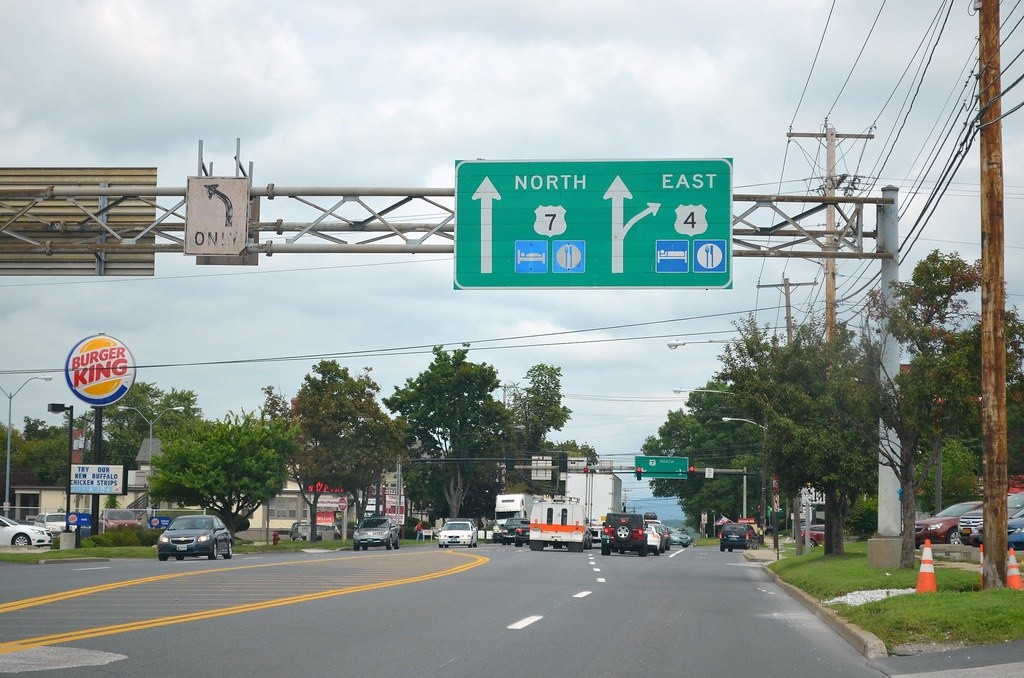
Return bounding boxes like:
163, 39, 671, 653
529, 494, 588, 551
493, 493, 527, 543
565, 467, 627, 542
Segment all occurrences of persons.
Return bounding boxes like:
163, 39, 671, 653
416, 521, 423, 541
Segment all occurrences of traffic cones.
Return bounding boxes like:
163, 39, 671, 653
1007, 548, 1024, 590
979, 545, 987, 592
915, 539, 938, 592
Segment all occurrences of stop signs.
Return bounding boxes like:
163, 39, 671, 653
338, 497, 348, 511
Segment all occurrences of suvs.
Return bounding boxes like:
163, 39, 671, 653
502, 518, 528, 545
601, 512, 648, 557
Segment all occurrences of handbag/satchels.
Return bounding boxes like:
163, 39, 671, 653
420, 530, 422, 533
413, 525, 418, 532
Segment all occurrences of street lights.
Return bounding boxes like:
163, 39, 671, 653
0, 375, 53, 518
117, 405, 185, 529
47, 403, 76, 550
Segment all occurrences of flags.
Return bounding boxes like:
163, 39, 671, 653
713, 515, 729, 526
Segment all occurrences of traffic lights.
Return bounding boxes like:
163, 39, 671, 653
636, 466, 642, 481
689, 466, 696, 478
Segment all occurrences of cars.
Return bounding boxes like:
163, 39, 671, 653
438, 517, 478, 548
958, 492, 1024, 550
34, 513, 80, 538
794, 524, 826, 547
352, 513, 400, 551
156, 515, 233, 560
288, 521, 340, 541
105, 508, 143, 531
644, 512, 692, 556
0, 516, 54, 546
515, 520, 530, 547
913, 501, 984, 545
719, 523, 759, 552
585, 525, 592, 549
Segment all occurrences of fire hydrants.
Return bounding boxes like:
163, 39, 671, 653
272, 528, 281, 547
